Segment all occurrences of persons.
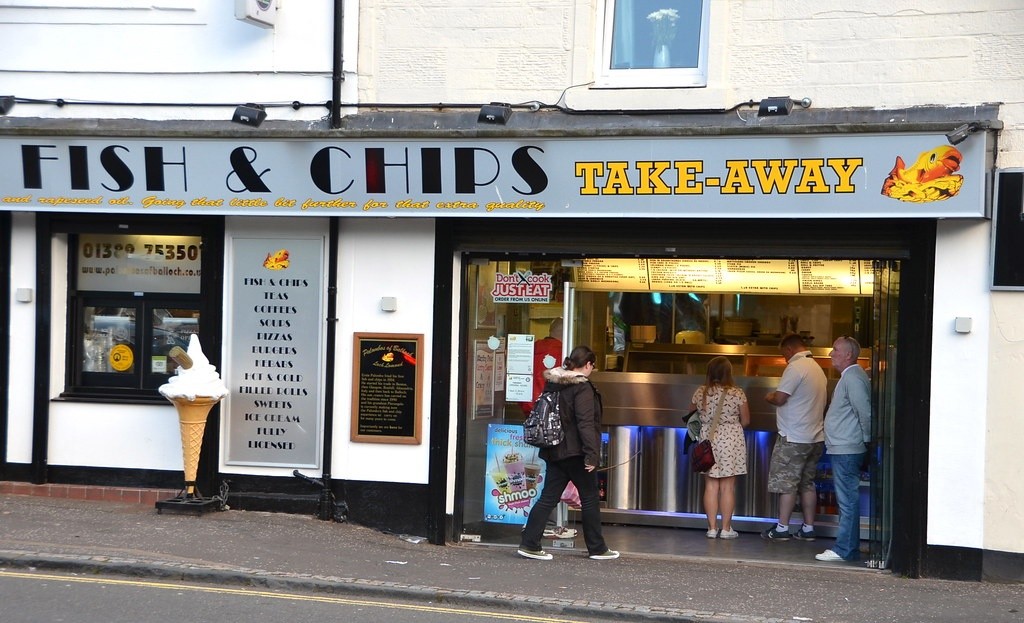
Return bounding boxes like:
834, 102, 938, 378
687, 356, 751, 540
761, 334, 828, 542
517, 346, 620, 559
816, 335, 870, 561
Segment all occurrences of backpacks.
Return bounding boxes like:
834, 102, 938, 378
523, 380, 573, 446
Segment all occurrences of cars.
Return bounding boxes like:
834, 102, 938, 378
83, 315, 200, 374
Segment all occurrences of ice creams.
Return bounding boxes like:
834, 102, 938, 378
159, 334, 228, 494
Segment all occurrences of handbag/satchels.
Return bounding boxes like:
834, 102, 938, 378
691, 439, 715, 472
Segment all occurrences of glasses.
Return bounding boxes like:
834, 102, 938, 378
842, 334, 854, 353
590, 362, 596, 370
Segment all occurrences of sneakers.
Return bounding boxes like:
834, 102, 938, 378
518, 548, 553, 560
815, 549, 845, 562
761, 522, 790, 541
542, 526, 578, 539
720, 526, 738, 539
706, 527, 719, 538
793, 522, 815, 541
589, 547, 619, 559
